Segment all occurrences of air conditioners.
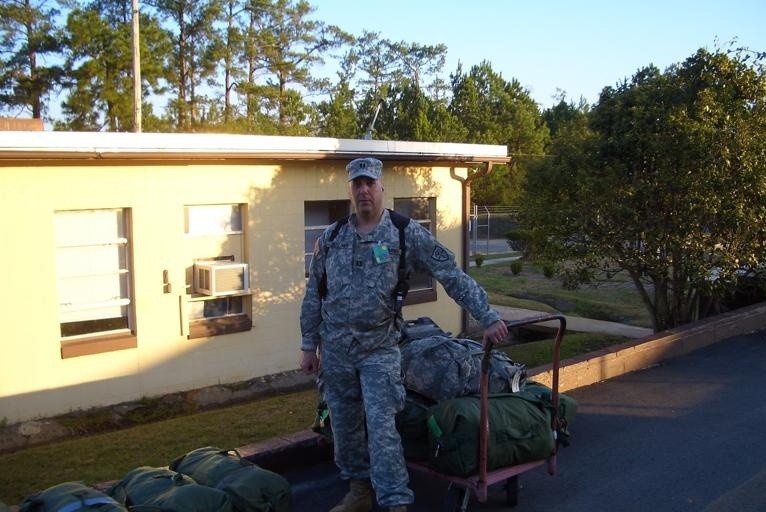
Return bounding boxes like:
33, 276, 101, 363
194, 261, 248, 297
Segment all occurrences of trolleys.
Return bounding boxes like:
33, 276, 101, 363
312, 312, 567, 509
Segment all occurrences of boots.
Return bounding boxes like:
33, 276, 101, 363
328, 478, 372, 512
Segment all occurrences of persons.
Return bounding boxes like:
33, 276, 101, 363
299, 158, 509, 512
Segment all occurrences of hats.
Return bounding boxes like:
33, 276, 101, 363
346, 158, 383, 182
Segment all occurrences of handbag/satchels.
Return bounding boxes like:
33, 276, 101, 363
169, 445, 291, 512
19, 481, 127, 512
428, 379, 577, 477
313, 390, 430, 463
109, 465, 234, 512
400, 317, 527, 399
